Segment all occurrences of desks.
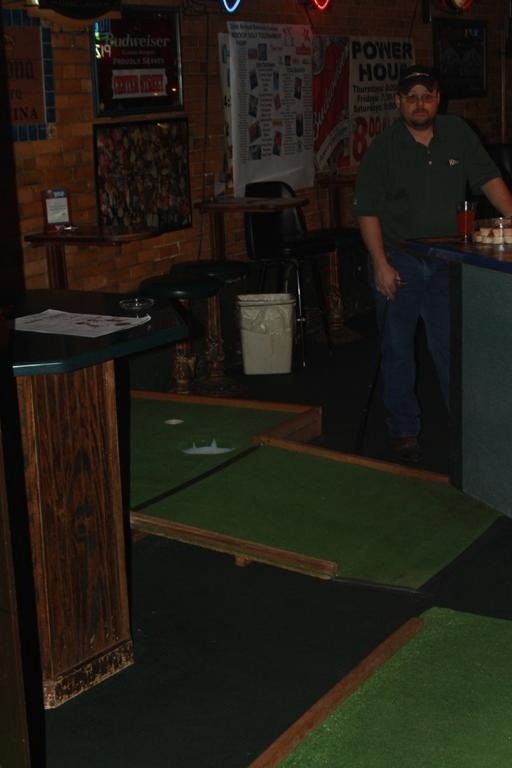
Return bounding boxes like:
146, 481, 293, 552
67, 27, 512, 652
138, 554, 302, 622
4, 287, 198, 712
400, 235, 512, 523
26, 219, 167, 293
197, 195, 312, 263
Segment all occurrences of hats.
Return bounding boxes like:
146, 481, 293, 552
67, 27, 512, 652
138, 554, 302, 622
396, 64, 434, 94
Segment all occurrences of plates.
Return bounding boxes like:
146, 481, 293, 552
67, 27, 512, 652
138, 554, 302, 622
120, 295, 158, 311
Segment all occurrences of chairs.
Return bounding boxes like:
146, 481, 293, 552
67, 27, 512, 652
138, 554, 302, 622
240, 177, 368, 381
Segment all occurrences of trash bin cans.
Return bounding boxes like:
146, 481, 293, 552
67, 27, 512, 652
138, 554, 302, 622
236, 259, 297, 375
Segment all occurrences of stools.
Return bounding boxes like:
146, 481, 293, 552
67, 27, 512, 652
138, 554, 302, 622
131, 250, 253, 401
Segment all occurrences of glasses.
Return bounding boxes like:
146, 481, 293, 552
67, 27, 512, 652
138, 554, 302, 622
407, 94, 439, 104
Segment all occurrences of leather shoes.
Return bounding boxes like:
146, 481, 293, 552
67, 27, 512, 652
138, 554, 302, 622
389, 435, 424, 464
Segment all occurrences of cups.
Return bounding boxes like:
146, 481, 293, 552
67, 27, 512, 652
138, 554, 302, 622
453, 198, 478, 244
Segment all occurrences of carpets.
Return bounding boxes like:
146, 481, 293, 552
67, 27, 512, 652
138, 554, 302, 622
131, 394, 503, 590
273, 604, 512, 767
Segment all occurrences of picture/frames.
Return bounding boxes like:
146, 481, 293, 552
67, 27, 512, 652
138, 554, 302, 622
91, 115, 194, 240
87, 3, 185, 117
429, 15, 490, 100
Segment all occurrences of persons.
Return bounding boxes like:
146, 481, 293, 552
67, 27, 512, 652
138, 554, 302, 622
352, 64, 512, 463
